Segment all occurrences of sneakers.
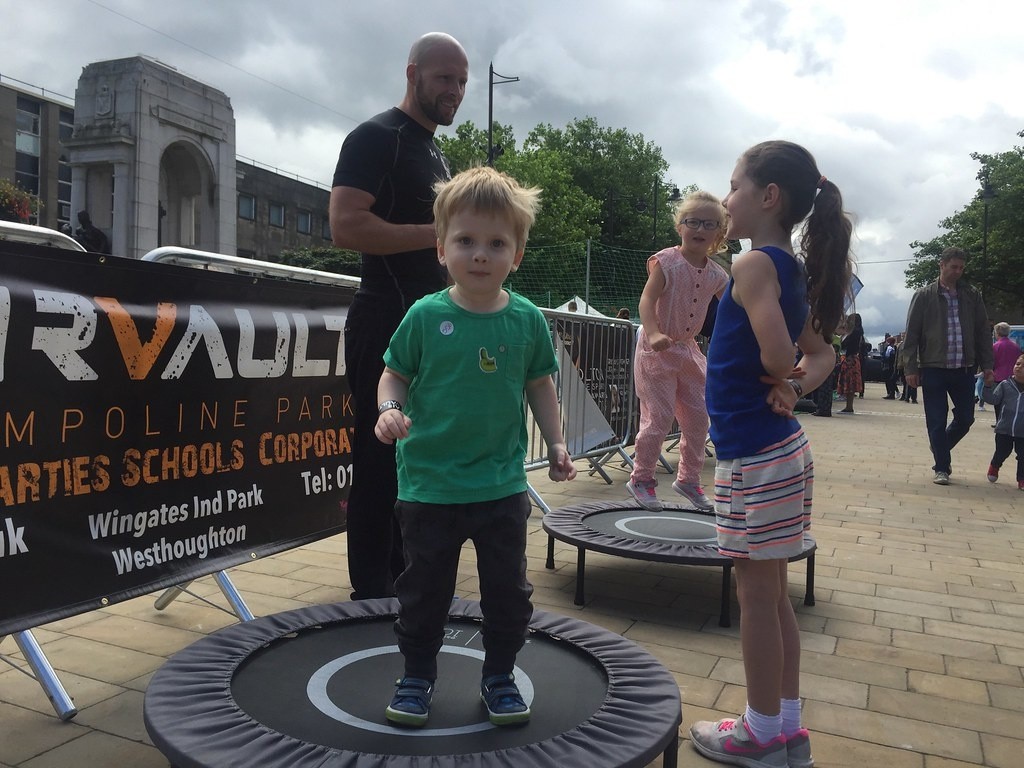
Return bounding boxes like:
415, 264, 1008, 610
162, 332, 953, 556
385, 675, 434, 728
836, 408, 855, 414
626, 478, 663, 512
672, 478, 715, 510
987, 462, 998, 482
1018, 480, 1024, 491
787, 730, 814, 767
688, 713, 787, 767
479, 672, 531, 726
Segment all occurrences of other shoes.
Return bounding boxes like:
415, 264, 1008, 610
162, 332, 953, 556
811, 409, 832, 417
859, 393, 864, 399
991, 424, 997, 428
882, 392, 919, 404
930, 443, 952, 476
933, 471, 949, 486
978, 405, 986, 412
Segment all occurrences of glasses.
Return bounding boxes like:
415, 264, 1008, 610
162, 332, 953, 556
677, 218, 720, 230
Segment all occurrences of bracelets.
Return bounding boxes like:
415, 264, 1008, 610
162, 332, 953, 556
379, 400, 401, 415
788, 379, 802, 397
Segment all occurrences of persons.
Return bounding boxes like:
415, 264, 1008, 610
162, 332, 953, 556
902, 247, 994, 484
981, 354, 1024, 491
328, 33, 469, 601
974, 366, 985, 411
991, 322, 1023, 427
617, 308, 629, 319
626, 190, 731, 511
690, 141, 852, 768
373, 166, 577, 726
76, 210, 108, 251
792, 312, 870, 417
568, 302, 577, 312
880, 331, 918, 403
